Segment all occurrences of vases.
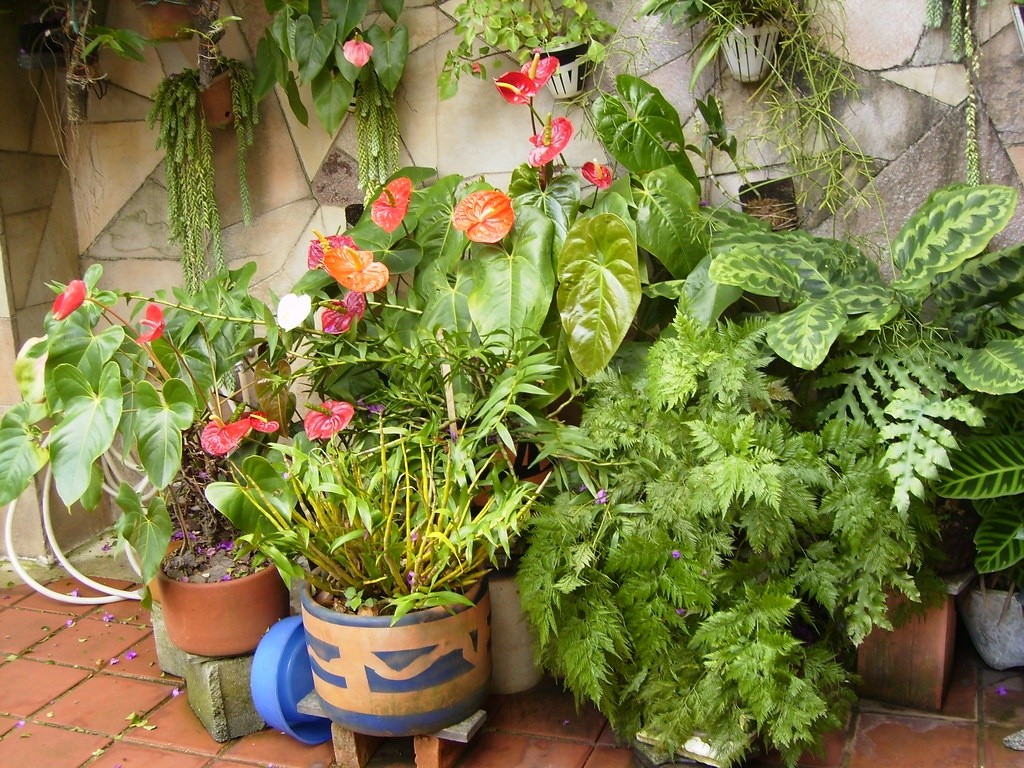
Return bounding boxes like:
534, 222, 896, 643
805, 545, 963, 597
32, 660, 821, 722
486, 574, 549, 694
157, 536, 291, 658
326, 55, 393, 115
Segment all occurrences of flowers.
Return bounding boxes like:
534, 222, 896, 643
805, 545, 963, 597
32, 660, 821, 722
302, 52, 646, 503
0, 263, 359, 592
254, 0, 411, 140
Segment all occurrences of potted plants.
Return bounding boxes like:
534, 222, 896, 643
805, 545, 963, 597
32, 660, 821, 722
148, 57, 260, 289
931, 384, 1024, 670
438, 0, 615, 98
635, 0, 831, 82
705, 181, 1024, 714
226, 441, 550, 736
